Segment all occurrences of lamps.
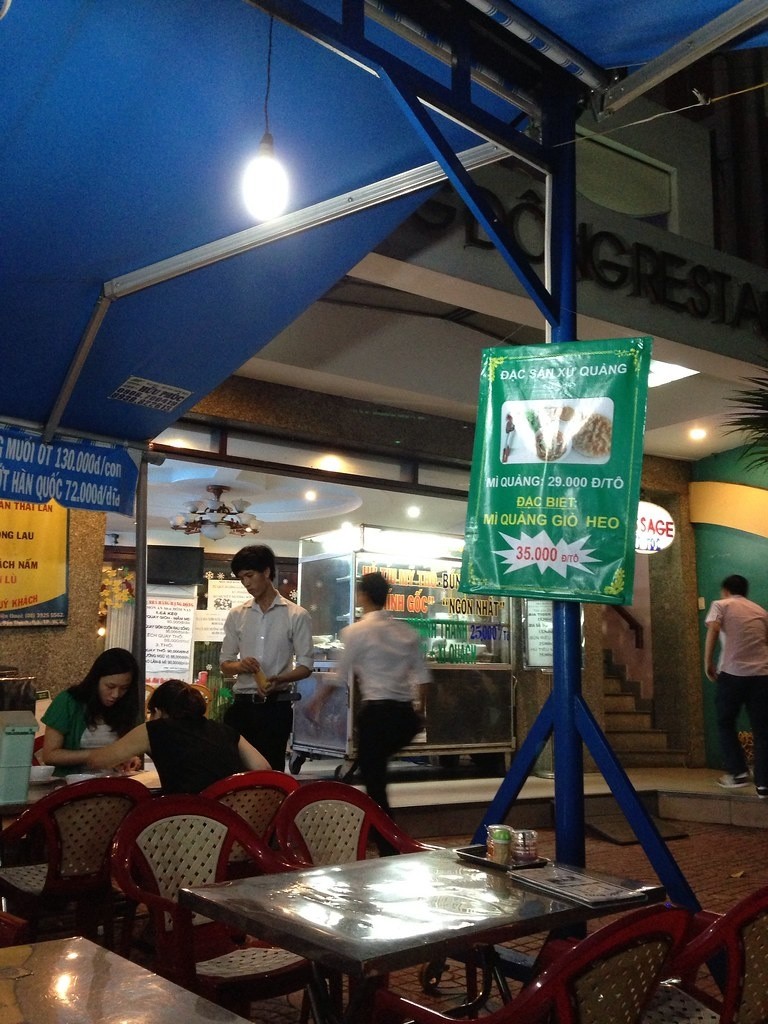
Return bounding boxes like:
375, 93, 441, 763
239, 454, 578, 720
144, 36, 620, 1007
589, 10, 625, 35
167, 483, 265, 544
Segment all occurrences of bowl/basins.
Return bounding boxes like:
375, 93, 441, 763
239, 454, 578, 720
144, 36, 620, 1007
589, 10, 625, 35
30, 766, 55, 784
66, 773, 97, 785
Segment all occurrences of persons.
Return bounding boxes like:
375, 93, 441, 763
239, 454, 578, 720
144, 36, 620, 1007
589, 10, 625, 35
220, 546, 314, 771
88, 680, 273, 795
705, 574, 768, 800
40, 648, 143, 774
303, 572, 433, 855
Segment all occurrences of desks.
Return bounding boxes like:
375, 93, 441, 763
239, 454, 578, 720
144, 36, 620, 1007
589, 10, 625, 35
0, 936, 256, 1024
178, 843, 668, 1024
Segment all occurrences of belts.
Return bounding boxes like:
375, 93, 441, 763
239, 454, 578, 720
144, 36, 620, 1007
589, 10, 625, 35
235, 692, 302, 703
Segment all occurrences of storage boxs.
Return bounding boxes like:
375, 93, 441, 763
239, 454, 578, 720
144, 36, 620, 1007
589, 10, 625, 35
0, 711, 39, 806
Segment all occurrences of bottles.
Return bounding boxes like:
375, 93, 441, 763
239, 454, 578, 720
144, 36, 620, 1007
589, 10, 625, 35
486, 825, 514, 867
513, 829, 539, 866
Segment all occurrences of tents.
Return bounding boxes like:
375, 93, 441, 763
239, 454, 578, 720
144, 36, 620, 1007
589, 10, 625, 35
0, 0, 768, 1024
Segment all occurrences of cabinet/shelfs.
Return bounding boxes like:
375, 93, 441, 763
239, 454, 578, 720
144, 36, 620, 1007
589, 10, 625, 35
290, 523, 516, 781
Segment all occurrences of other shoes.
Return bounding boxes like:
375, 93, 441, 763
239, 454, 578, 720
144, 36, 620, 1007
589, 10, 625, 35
756, 787, 768, 799
717, 775, 751, 788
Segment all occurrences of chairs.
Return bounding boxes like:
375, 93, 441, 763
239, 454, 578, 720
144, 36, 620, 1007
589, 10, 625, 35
0, 766, 768, 1024
